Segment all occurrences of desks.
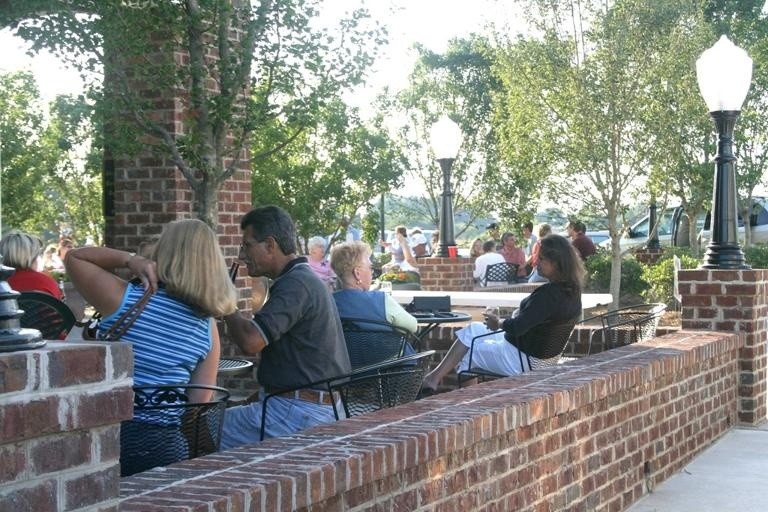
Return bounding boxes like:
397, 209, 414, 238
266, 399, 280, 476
380, 294, 469, 340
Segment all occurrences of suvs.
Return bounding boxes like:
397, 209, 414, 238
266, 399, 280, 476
595, 193, 768, 252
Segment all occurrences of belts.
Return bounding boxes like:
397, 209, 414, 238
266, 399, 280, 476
265, 387, 339, 405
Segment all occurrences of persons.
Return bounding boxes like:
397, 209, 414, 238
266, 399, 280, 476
305, 236, 337, 285
205, 205, 351, 452
67, 219, 239, 478
0, 233, 66, 340
469, 221, 595, 287
340, 215, 359, 242
331, 241, 417, 378
421, 234, 588, 391
378, 226, 439, 274
53, 240, 72, 268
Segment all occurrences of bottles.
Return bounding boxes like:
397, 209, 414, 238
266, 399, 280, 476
229, 261, 239, 284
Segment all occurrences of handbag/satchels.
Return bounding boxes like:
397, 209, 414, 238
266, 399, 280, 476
406, 297, 450, 311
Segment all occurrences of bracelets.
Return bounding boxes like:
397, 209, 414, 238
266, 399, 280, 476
125, 252, 136, 267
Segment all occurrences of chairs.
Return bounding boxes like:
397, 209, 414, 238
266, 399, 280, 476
457, 313, 571, 382
567, 300, 667, 365
326, 315, 423, 369
480, 261, 521, 294
20, 254, 257, 482
250, 347, 442, 437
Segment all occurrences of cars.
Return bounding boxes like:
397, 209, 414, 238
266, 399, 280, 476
354, 226, 444, 256
551, 227, 614, 251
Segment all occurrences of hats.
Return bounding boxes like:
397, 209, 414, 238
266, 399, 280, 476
486, 223, 499, 229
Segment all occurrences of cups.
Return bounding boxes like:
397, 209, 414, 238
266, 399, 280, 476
447, 246, 458, 257
483, 304, 502, 321
379, 280, 394, 295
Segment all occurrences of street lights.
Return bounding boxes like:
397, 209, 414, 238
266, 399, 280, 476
426, 110, 464, 258
689, 30, 757, 269
